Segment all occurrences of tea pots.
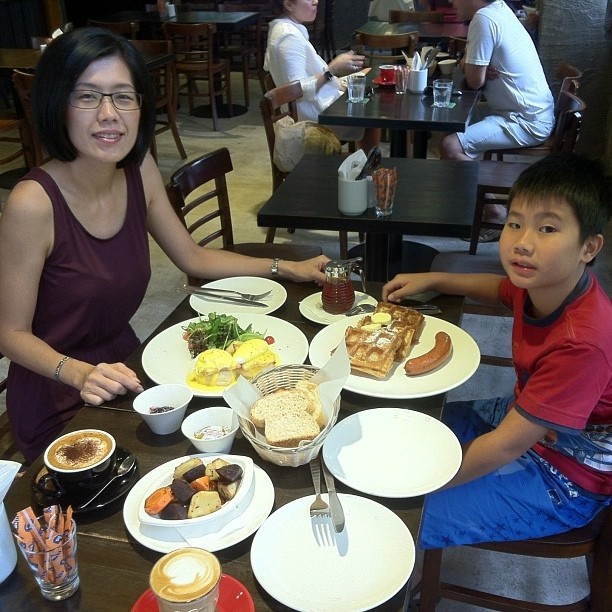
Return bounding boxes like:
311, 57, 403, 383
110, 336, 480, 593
321, 260, 355, 315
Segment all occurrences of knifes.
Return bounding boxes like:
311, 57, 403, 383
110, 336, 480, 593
322, 457, 345, 533
188, 291, 269, 307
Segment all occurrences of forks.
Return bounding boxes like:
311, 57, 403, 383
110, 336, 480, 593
345, 303, 437, 317
187, 286, 272, 300
308, 457, 332, 518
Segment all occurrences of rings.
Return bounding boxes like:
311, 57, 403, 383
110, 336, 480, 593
353, 65, 359, 71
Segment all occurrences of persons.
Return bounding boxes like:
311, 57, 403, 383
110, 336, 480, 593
1, 25, 331, 469
437, 0, 558, 243
380, 154, 611, 550
263, 1, 382, 155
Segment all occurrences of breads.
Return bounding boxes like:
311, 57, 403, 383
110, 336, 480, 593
252, 380, 324, 444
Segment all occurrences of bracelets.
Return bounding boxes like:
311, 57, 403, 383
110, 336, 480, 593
269, 257, 284, 282
55, 355, 70, 390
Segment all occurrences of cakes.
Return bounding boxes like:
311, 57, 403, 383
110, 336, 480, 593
186, 339, 280, 391
331, 302, 424, 378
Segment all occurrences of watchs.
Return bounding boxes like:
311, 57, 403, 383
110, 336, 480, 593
322, 65, 335, 85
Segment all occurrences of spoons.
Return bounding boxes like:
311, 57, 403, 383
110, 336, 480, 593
77, 454, 136, 509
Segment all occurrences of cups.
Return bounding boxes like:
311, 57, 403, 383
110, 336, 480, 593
427, 60, 437, 76
181, 406, 240, 453
365, 175, 375, 209
438, 59, 457, 74
133, 384, 194, 435
337, 174, 367, 216
373, 168, 397, 217
347, 76, 366, 103
18, 517, 80, 601
408, 68, 428, 94
149, 547, 222, 612
37, 429, 117, 499
433, 79, 453, 107
379, 65, 395, 82
393, 65, 410, 95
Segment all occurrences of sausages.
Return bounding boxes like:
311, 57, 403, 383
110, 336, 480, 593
405, 331, 452, 375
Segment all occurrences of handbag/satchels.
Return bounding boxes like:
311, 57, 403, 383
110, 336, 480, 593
273, 115, 341, 172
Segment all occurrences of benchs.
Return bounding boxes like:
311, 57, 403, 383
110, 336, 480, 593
449, 35, 467, 60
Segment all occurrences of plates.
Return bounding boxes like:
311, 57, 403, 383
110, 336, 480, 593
299, 289, 380, 325
190, 275, 288, 315
309, 311, 481, 400
31, 449, 147, 514
141, 315, 308, 398
371, 77, 396, 87
250, 493, 416, 611
129, 573, 254, 612
321, 406, 463, 498
139, 457, 254, 526
122, 452, 275, 554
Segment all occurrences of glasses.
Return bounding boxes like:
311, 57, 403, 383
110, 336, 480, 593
68, 88, 143, 111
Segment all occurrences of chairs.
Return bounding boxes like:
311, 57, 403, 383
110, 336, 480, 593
483, 63, 582, 159
389, 10, 442, 23
166, 147, 322, 286
169, 23, 231, 129
144, 53, 187, 167
313, 1, 337, 61
469, 92, 587, 254
260, 80, 364, 256
411, 507, 612, 612
483, 81, 580, 160
0, 353, 27, 466
86, 17, 141, 40
0, 119, 29, 176
433, 251, 515, 369
449, 36, 467, 66
13, 70, 53, 167
354, 30, 419, 65
